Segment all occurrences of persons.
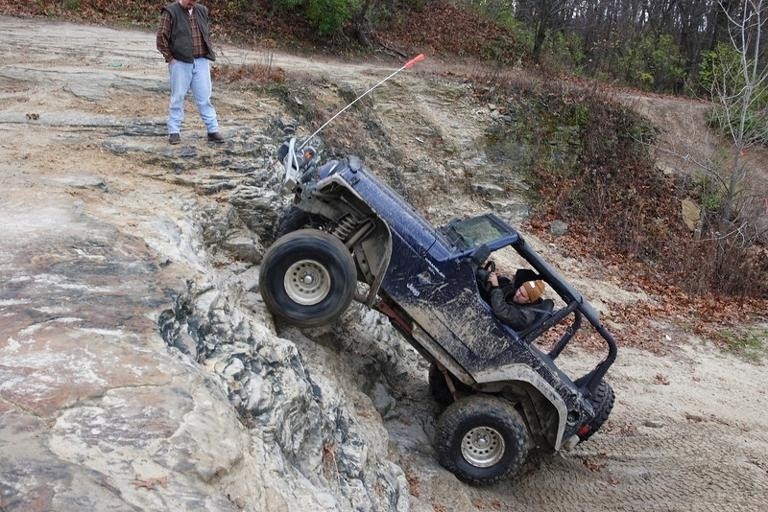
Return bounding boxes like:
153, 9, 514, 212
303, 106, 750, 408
483, 270, 545, 329
155, 0, 224, 143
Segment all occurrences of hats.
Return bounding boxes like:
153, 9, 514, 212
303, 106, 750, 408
522, 280, 545, 303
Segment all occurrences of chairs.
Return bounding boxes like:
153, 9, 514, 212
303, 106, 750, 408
516, 299, 555, 339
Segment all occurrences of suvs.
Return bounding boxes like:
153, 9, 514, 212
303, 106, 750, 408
258, 136, 618, 486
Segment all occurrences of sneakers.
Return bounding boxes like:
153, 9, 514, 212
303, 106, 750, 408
208, 132, 224, 142
169, 133, 181, 144
